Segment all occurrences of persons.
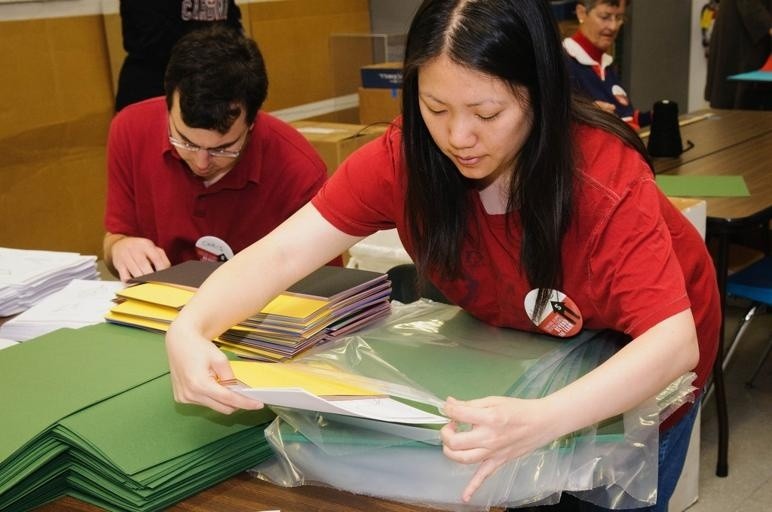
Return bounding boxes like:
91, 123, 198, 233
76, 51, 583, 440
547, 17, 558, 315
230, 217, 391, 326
561, 0, 656, 133
104, 22, 343, 284
705, 0, 772, 110
116, 0, 242, 112
699, 0, 717, 58
162, 0, 723, 512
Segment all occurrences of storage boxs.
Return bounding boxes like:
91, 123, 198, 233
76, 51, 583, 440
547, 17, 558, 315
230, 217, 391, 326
289, 60, 405, 268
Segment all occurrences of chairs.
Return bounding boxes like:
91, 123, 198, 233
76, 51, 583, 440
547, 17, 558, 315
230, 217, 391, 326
703, 251, 771, 408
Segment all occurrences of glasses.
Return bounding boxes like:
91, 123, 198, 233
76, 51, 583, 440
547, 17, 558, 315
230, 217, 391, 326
168, 127, 249, 157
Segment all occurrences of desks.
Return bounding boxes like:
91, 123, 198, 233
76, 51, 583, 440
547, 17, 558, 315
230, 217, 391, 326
636, 108, 772, 478
